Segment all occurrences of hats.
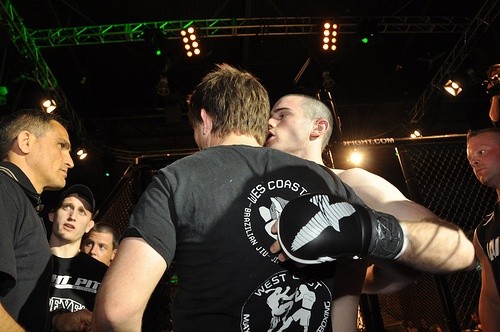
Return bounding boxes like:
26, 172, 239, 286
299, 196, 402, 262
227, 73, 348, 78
56, 183, 96, 212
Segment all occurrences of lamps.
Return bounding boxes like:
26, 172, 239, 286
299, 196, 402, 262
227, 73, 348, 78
443, 78, 463, 97
180, 25, 202, 60
319, 21, 340, 54
410, 130, 424, 139
74, 144, 89, 161
40, 95, 58, 114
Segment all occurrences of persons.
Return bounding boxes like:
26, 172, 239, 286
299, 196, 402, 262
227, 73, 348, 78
82, 223, 121, 265
0, 110, 74, 332
260, 92, 476, 332
455, 121, 500, 332
93, 59, 368, 332
40, 185, 113, 331
